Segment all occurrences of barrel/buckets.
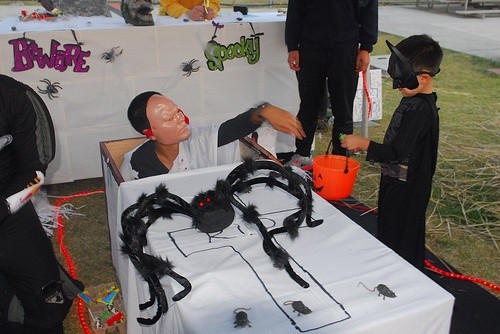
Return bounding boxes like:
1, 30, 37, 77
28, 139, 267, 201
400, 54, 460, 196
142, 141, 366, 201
313, 139, 360, 201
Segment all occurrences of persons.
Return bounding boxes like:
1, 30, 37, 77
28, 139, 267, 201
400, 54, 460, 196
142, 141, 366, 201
0, 74, 64, 334
340, 34, 444, 273
120, 91, 306, 182
283, 0, 379, 167
157, 0, 221, 21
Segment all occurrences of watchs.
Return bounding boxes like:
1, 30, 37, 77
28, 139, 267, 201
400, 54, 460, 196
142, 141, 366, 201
256, 102, 271, 119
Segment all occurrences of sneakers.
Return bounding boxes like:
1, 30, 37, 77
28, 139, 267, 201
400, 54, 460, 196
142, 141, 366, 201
285, 154, 313, 172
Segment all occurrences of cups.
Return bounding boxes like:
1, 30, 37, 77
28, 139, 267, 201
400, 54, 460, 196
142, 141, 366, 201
234, 6, 248, 15
276, 8, 287, 15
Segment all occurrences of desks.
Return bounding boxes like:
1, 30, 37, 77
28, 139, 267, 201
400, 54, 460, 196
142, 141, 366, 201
0, 7, 315, 184
